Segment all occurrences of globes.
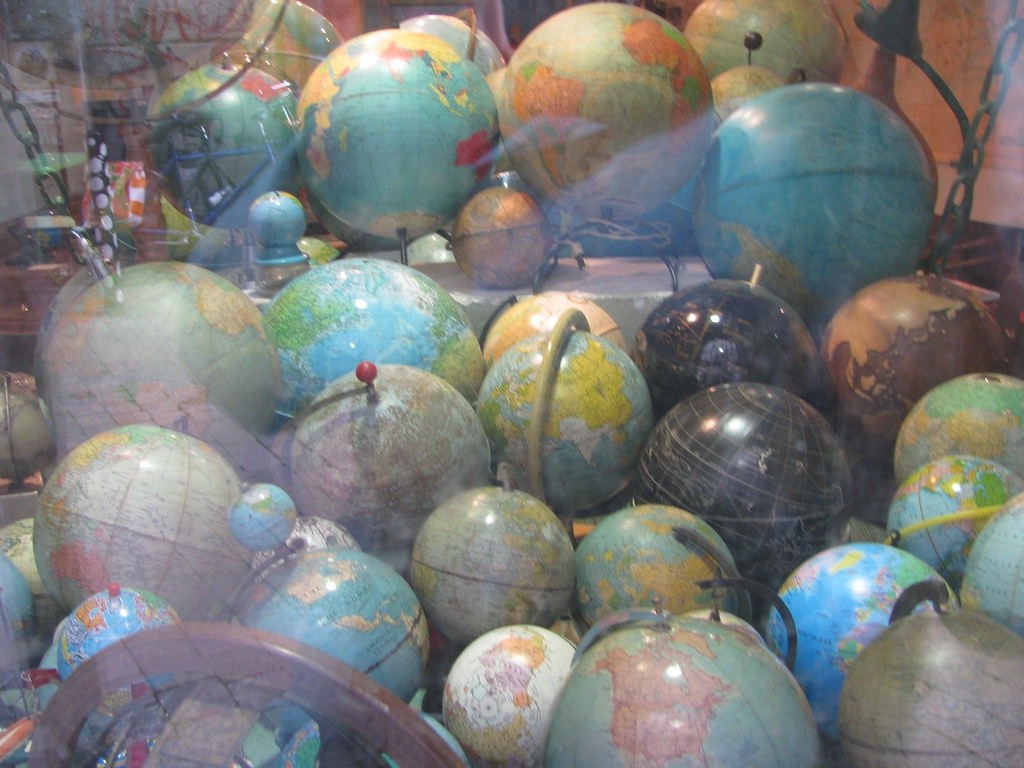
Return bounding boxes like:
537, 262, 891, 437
683, 0, 846, 123
0, 0, 344, 299
693, 69, 936, 332
396, 7, 507, 74
0, 260, 1023, 767
295, 28, 499, 265
444, 187, 559, 289
496, 0, 716, 294
143, 50, 303, 283
